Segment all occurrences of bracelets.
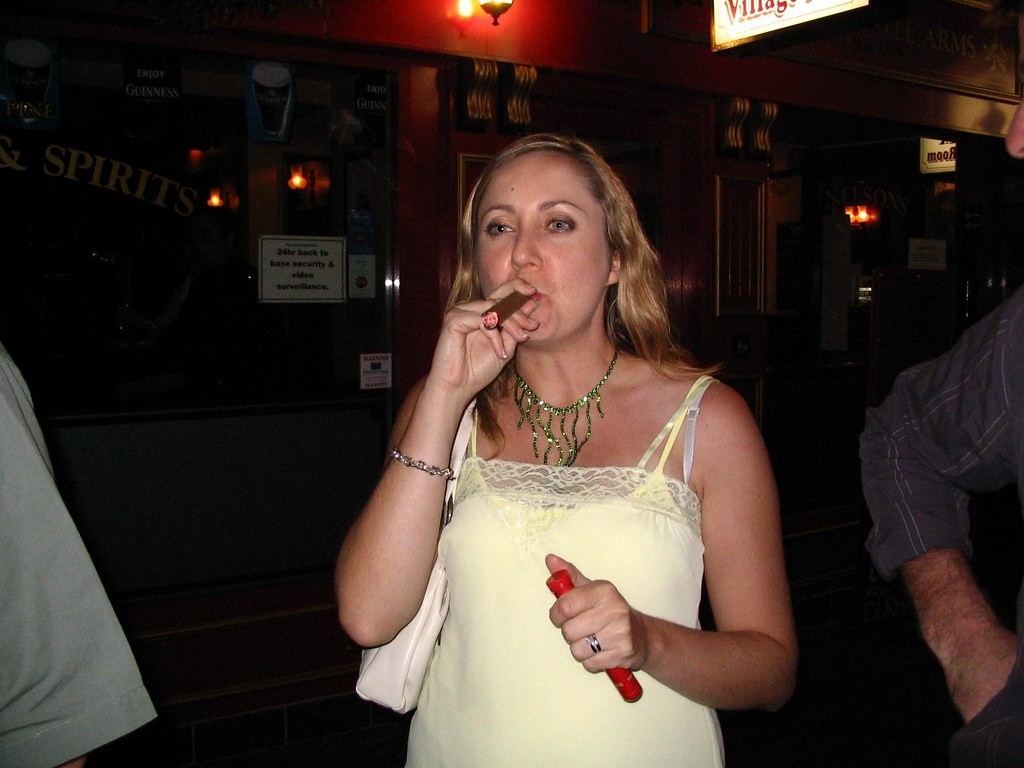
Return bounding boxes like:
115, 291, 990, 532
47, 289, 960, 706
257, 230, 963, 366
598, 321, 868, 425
388, 445, 455, 481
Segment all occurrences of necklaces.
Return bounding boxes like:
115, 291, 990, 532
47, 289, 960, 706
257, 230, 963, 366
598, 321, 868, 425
515, 345, 619, 467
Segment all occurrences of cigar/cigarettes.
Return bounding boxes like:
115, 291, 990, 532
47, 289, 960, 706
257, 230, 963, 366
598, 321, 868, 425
480, 292, 534, 330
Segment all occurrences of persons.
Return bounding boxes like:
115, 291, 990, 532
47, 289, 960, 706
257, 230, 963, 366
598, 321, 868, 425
0, 344, 157, 767
858, 98, 1024, 768
332, 134, 799, 768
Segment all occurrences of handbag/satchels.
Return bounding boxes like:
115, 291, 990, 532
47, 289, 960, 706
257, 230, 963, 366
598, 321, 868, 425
355, 399, 477, 715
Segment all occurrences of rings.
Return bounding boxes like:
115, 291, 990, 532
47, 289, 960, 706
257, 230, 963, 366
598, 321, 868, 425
585, 634, 602, 653
